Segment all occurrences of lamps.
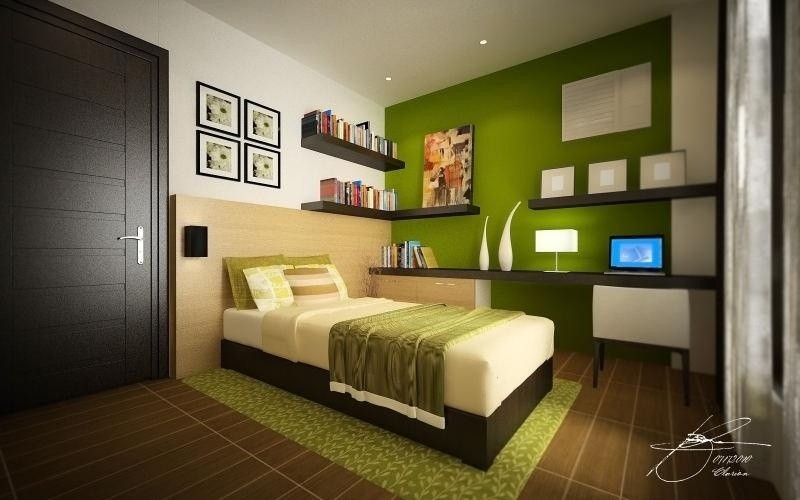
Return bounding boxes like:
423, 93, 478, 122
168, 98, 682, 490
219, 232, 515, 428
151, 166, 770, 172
185, 226, 207, 257
536, 228, 578, 274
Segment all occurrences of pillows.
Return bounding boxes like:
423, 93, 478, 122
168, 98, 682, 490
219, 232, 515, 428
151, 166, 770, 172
283, 263, 350, 297
279, 254, 349, 297
224, 254, 285, 309
284, 268, 340, 301
242, 264, 295, 312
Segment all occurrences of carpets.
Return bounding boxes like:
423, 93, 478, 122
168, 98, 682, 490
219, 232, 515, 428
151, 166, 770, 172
182, 368, 583, 500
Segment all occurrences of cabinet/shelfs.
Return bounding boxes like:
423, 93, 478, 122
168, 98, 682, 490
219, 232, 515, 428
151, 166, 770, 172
301, 128, 482, 220
372, 274, 491, 312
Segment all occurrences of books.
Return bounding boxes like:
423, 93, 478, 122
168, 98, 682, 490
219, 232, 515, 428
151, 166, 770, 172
303, 109, 398, 160
381, 240, 438, 271
320, 178, 398, 212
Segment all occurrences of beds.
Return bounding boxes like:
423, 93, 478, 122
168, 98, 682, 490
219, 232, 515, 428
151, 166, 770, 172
221, 298, 555, 470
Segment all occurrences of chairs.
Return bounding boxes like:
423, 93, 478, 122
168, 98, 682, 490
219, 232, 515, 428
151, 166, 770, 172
590, 285, 693, 404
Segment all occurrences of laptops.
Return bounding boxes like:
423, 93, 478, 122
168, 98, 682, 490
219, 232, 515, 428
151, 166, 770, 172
603, 234, 667, 277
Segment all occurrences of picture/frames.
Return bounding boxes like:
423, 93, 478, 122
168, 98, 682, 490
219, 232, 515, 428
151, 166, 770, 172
243, 143, 280, 188
244, 99, 280, 148
196, 81, 240, 137
196, 131, 240, 181
